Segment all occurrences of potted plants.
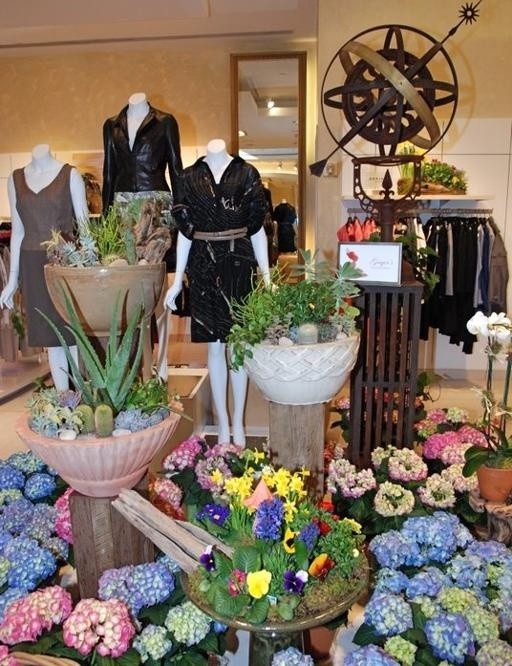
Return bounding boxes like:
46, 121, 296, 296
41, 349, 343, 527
37, 197, 183, 338
17, 277, 193, 500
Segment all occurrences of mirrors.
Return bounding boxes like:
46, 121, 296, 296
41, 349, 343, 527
230, 49, 307, 284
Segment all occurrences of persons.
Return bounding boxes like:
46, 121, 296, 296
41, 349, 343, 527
101, 89, 183, 386
1, 142, 98, 395
163, 137, 281, 450
272, 199, 298, 252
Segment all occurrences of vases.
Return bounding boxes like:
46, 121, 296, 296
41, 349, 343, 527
425, 180, 466, 195
239, 328, 362, 406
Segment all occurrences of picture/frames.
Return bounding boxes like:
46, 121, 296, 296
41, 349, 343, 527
336, 240, 403, 287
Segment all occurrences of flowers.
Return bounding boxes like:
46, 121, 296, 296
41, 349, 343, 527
223, 247, 369, 373
421, 157, 468, 191
0, 309, 512, 666
